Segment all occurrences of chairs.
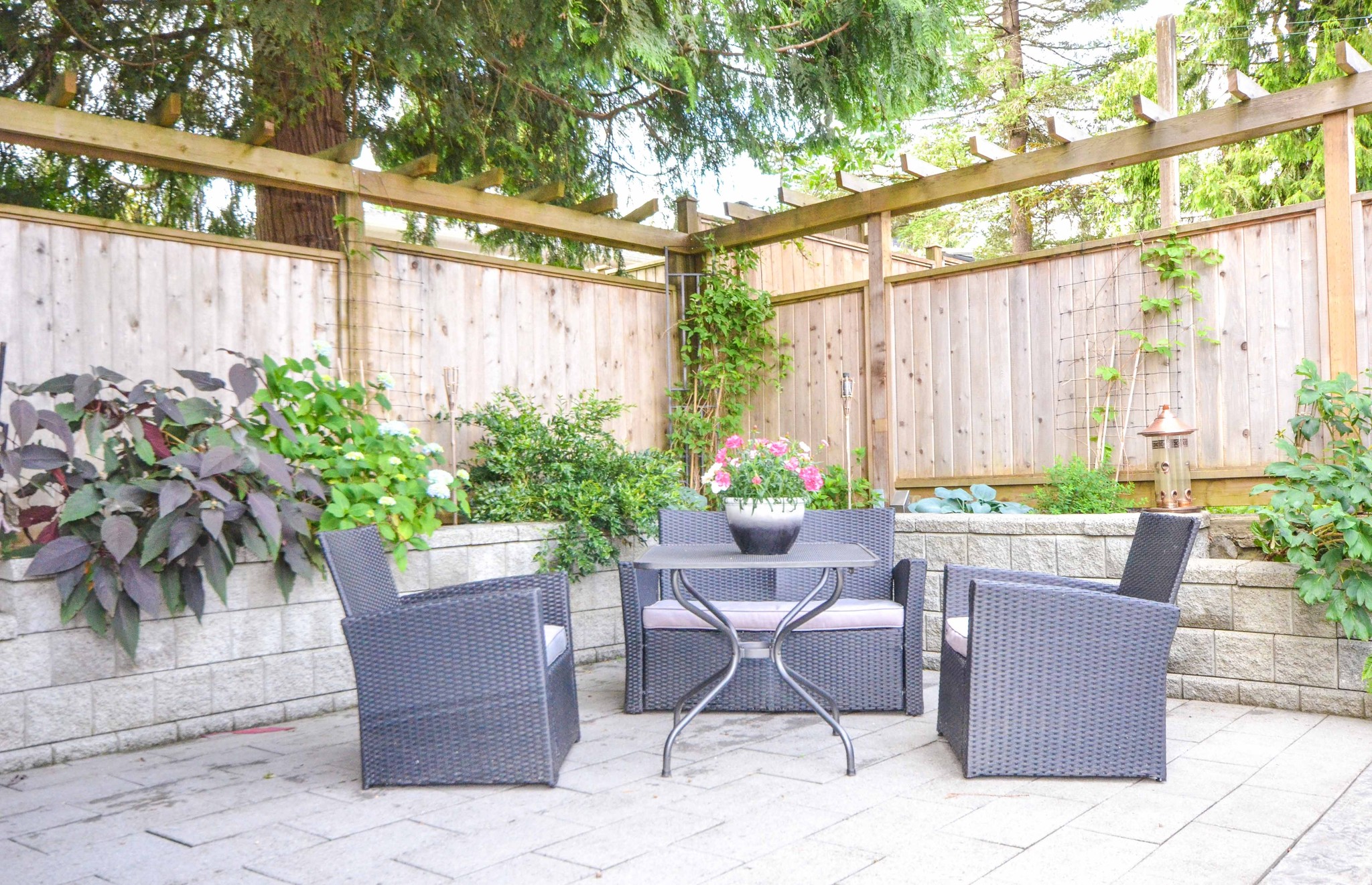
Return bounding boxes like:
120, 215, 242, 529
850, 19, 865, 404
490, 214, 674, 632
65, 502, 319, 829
937, 512, 1202, 780
320, 525, 582, 790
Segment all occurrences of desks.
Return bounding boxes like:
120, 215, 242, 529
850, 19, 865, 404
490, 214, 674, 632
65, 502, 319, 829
633, 540, 879, 779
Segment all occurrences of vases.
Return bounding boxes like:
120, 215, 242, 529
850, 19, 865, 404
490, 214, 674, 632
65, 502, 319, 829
617, 510, 927, 716
723, 496, 805, 554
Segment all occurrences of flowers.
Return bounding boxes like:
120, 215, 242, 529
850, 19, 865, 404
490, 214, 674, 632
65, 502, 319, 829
700, 436, 825, 513
